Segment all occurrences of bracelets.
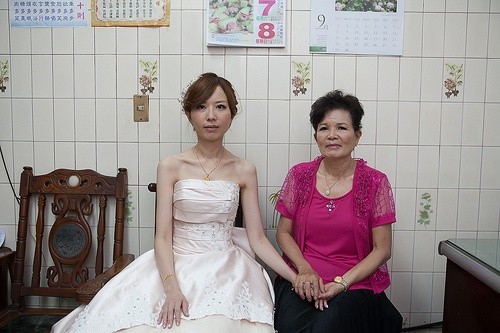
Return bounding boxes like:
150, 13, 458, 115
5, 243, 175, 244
162, 272, 176, 281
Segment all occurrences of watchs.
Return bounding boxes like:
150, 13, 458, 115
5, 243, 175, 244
334, 276, 350, 292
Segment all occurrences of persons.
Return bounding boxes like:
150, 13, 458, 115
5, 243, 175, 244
275, 89, 404, 333
50, 73, 329, 333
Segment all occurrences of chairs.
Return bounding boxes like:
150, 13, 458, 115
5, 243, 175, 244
0, 166, 134, 333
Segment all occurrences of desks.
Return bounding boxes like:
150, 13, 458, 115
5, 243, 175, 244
435, 238, 500, 333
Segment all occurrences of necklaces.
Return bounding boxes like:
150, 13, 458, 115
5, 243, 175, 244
325, 175, 340, 195
196, 148, 221, 181
